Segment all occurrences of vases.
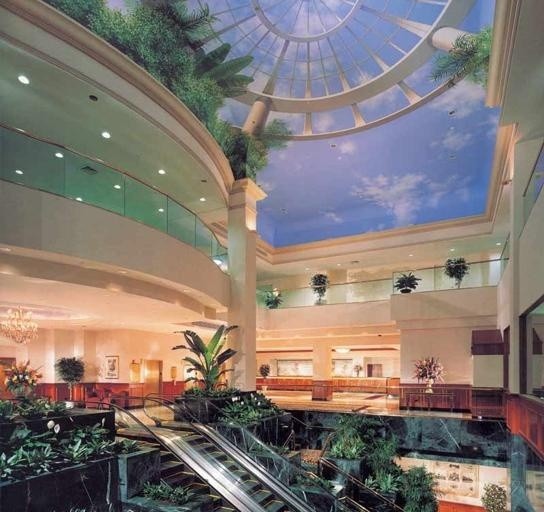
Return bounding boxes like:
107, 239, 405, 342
11, 387, 32, 398
424, 378, 434, 393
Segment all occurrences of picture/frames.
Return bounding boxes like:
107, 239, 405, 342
104, 356, 119, 379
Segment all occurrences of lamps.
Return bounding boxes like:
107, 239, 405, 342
2, 308, 38, 343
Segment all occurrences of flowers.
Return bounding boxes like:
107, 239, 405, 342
412, 357, 445, 382
3, 359, 43, 393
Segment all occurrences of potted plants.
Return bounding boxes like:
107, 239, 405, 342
54, 357, 85, 409
310, 274, 330, 305
259, 363, 270, 391
265, 294, 283, 309
394, 272, 422, 293
